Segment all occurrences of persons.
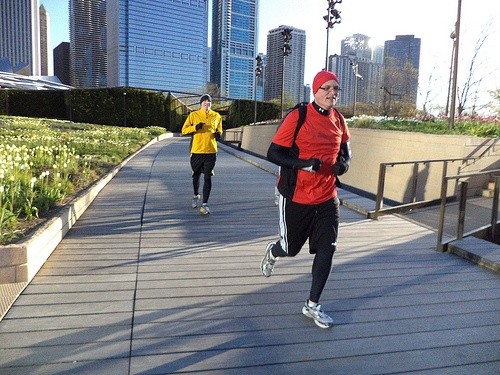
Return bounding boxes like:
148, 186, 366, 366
260, 70, 351, 328
182, 94, 223, 215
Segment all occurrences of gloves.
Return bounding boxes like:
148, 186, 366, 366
332, 156, 349, 176
293, 158, 323, 172
196, 122, 205, 130
214, 132, 220, 141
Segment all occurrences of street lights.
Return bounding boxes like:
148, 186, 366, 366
322, 0, 343, 71
123, 92, 127, 127
350, 63, 363, 116
280, 28, 293, 119
380, 86, 403, 116
253, 56, 263, 125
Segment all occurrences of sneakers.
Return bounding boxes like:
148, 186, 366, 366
302, 299, 334, 328
200, 203, 210, 214
191, 195, 200, 208
260, 241, 277, 277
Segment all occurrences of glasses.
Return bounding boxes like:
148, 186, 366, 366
319, 86, 342, 91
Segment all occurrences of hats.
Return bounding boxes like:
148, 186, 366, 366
312, 71, 339, 97
200, 94, 211, 105
